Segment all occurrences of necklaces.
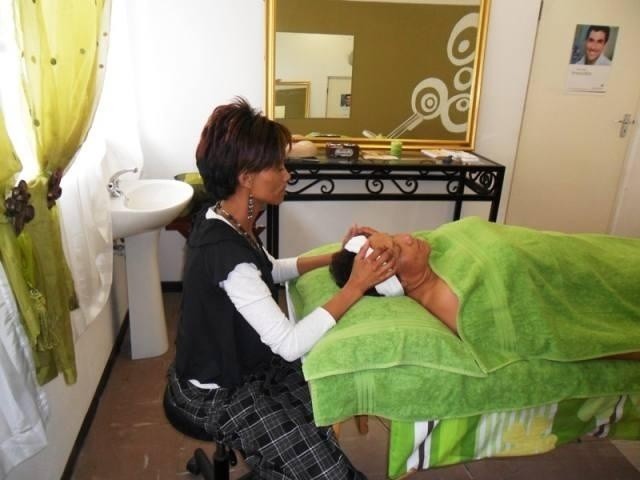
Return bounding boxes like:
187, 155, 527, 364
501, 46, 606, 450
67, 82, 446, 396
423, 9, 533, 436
216, 199, 264, 256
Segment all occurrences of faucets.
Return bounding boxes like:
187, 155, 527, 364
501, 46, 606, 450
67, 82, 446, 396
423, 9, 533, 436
107, 167, 138, 195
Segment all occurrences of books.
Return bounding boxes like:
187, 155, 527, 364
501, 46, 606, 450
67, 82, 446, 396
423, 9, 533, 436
419, 149, 454, 160
447, 150, 478, 162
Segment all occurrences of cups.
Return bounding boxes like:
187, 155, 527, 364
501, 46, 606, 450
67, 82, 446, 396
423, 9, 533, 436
391, 142, 402, 157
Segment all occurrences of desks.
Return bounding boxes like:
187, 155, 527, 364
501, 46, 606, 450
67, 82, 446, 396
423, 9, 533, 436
265, 152, 506, 303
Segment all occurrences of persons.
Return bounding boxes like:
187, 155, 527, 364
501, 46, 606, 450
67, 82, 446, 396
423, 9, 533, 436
343, 94, 351, 107
161, 101, 399, 479
328, 231, 461, 336
573, 24, 614, 66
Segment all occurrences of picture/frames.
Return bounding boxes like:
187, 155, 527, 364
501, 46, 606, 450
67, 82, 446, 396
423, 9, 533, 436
325, 75, 352, 120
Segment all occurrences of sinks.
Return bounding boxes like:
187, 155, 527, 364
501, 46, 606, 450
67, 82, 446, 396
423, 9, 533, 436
110, 179, 194, 241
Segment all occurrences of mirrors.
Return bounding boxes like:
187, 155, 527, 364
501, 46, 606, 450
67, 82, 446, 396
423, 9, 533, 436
258, 0, 488, 152
275, 32, 355, 122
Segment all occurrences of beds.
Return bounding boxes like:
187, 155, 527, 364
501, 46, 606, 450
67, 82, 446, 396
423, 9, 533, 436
279, 247, 640, 480
